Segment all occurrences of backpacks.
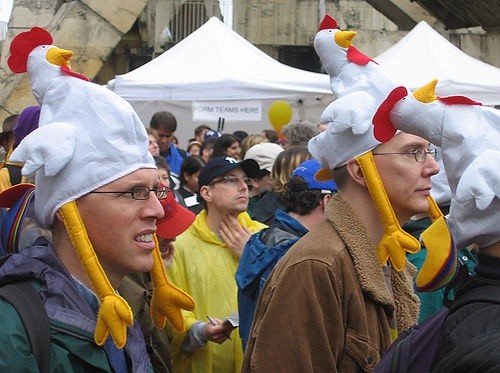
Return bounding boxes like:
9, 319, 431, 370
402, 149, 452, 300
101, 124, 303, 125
373, 265, 500, 373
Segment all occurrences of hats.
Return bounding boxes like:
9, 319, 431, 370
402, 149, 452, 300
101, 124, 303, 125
243, 142, 286, 172
8, 24, 197, 353
0, 113, 22, 148
204, 130, 224, 141
288, 160, 336, 189
306, 13, 444, 274
372, 75, 499, 250
198, 154, 259, 189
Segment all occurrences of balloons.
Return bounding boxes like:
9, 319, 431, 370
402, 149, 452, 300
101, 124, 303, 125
267, 100, 293, 132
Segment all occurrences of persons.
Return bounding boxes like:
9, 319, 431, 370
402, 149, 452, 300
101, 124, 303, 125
372, 79, 500, 373
0, 105, 478, 373
0, 27, 196, 373
236, 13, 441, 373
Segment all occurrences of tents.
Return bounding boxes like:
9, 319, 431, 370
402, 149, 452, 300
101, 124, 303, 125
101, 15, 500, 108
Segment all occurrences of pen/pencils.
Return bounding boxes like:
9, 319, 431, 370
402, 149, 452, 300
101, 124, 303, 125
207, 315, 233, 342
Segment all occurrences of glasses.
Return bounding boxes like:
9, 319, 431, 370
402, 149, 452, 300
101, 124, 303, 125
216, 176, 256, 186
373, 147, 443, 164
90, 184, 171, 201
